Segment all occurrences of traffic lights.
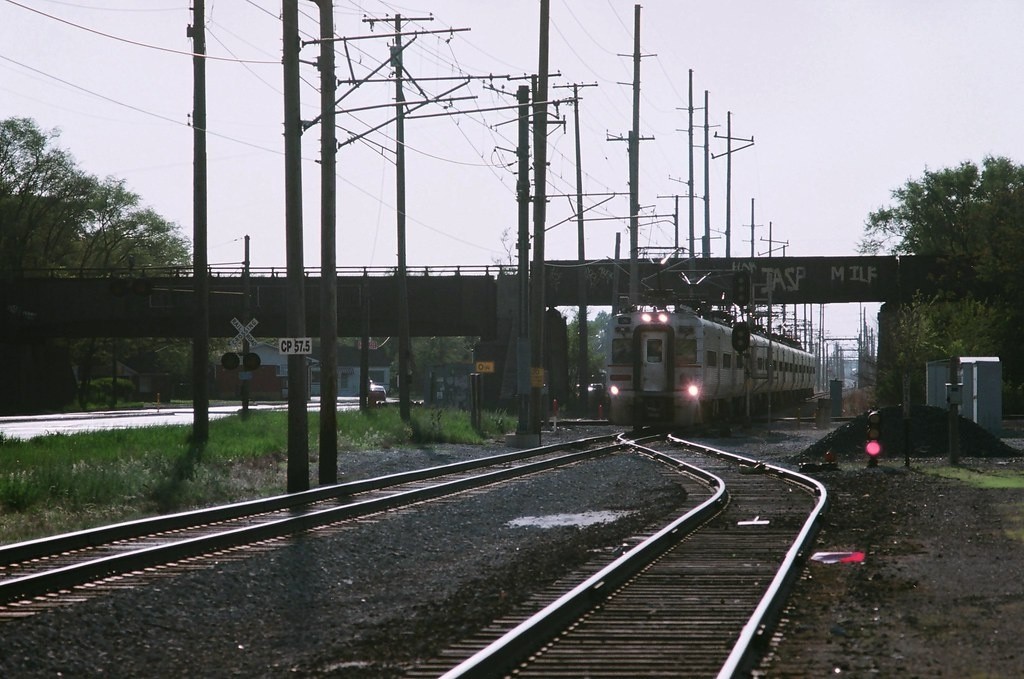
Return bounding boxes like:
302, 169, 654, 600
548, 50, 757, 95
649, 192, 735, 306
864, 409, 881, 468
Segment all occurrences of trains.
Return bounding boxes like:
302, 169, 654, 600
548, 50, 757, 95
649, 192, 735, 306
605, 313, 816, 435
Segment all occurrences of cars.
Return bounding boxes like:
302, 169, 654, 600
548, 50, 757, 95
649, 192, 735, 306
369, 386, 386, 401
